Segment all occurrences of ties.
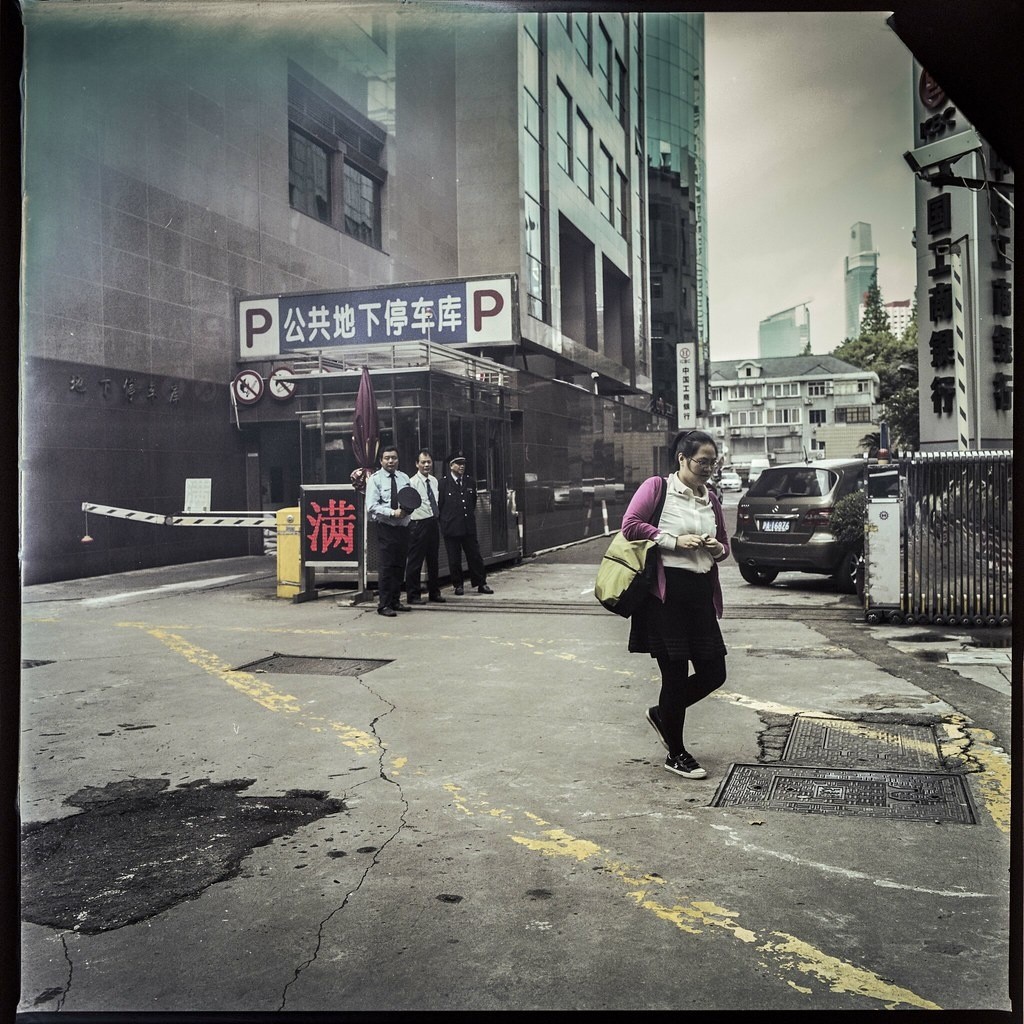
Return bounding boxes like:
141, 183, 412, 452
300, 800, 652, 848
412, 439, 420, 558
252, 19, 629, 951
389, 473, 398, 510
426, 479, 440, 518
456, 477, 461, 486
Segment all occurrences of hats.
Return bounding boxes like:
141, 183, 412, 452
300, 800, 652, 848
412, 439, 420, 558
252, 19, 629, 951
397, 487, 422, 512
446, 449, 466, 467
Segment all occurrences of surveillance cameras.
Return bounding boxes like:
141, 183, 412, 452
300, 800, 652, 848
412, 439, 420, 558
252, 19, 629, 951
902, 128, 981, 173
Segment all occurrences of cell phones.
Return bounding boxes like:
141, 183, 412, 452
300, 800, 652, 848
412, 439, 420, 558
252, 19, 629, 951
701, 532, 710, 539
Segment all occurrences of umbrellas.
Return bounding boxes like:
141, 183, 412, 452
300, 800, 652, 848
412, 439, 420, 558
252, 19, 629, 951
349, 365, 380, 590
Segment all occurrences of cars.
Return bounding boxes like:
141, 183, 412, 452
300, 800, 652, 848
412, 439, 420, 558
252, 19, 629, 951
720, 473, 743, 493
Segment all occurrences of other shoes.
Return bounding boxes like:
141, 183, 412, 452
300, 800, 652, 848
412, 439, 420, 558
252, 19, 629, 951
430, 595, 445, 602
455, 587, 464, 595
391, 603, 412, 611
478, 586, 493, 594
379, 608, 397, 616
408, 598, 426, 605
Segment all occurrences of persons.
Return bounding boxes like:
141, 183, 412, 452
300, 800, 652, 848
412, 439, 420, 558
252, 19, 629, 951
364, 446, 414, 616
405, 449, 445, 604
623, 430, 729, 779
437, 450, 494, 594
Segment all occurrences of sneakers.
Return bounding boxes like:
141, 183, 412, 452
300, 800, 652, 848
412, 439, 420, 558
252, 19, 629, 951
646, 705, 672, 751
663, 749, 707, 779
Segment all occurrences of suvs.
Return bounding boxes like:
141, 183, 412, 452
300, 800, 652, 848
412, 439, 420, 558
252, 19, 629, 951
731, 459, 869, 596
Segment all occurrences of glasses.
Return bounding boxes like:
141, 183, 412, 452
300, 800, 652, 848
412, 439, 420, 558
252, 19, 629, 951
682, 452, 717, 468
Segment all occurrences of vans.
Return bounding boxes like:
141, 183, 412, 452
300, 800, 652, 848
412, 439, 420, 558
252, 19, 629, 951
747, 459, 771, 489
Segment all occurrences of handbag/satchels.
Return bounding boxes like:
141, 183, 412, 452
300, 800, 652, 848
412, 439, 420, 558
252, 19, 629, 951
594, 476, 667, 619
